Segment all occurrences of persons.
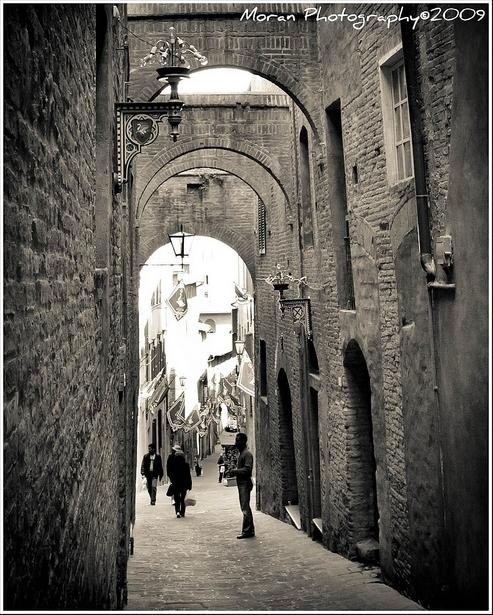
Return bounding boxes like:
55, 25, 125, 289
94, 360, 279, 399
140, 443, 163, 506
215, 453, 225, 483
224, 433, 255, 539
194, 455, 202, 477
170, 450, 192, 518
166, 444, 181, 505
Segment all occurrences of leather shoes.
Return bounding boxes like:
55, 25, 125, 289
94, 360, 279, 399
236, 532, 255, 539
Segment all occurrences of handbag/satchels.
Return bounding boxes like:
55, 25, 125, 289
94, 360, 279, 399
166, 482, 175, 497
184, 489, 197, 507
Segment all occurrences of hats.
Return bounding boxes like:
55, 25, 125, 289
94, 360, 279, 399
173, 444, 181, 451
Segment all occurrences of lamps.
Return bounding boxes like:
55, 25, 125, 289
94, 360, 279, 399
178, 375, 187, 393
233, 283, 249, 301
138, 224, 195, 270
264, 263, 313, 343
233, 335, 245, 366
112, 25, 208, 192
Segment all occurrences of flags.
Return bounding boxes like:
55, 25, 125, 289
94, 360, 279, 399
165, 279, 189, 323
182, 402, 222, 437
165, 391, 186, 433
234, 347, 255, 399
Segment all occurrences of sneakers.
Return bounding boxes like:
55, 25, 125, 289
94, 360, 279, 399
176, 511, 184, 518
